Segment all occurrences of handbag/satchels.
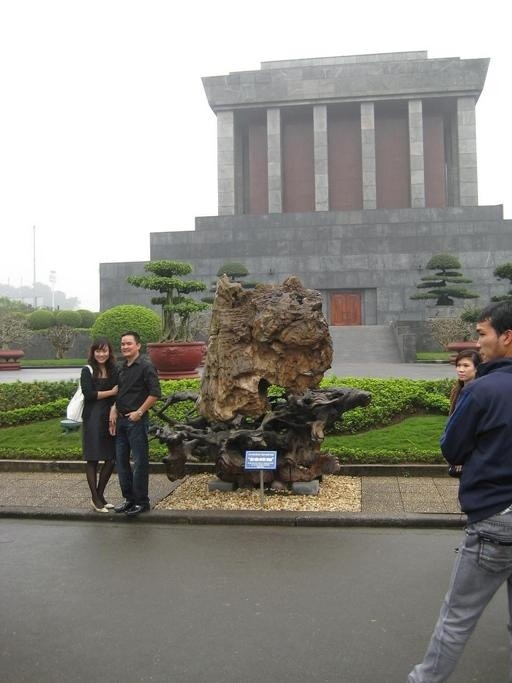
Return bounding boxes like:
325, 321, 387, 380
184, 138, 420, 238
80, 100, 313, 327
66, 364, 94, 422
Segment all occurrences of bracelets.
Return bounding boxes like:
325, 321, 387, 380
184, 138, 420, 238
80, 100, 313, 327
136, 409, 144, 417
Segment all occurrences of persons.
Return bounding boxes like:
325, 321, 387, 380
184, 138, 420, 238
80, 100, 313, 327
108, 331, 162, 517
405, 300, 512, 683
80, 337, 119, 513
449, 350, 482, 417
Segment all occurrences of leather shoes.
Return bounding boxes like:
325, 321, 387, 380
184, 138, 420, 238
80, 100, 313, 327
127, 503, 150, 515
114, 497, 134, 512
90, 498, 109, 512
104, 502, 115, 508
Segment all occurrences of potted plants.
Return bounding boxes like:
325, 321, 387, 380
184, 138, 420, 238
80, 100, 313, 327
410, 253, 479, 318
127, 259, 209, 381
